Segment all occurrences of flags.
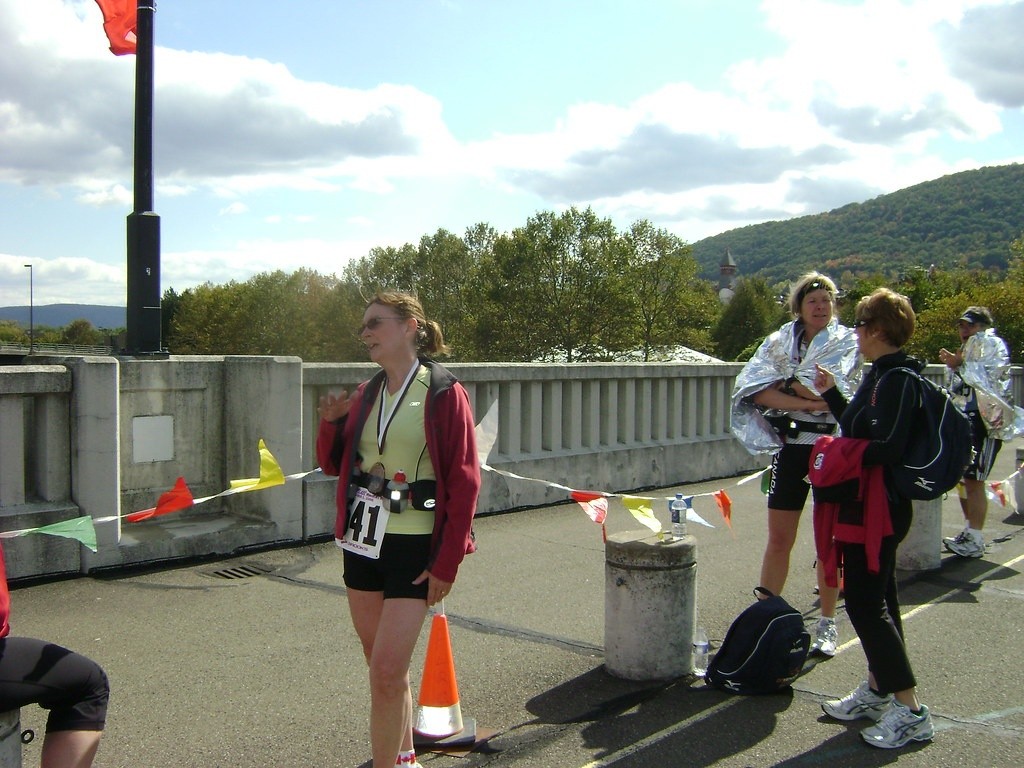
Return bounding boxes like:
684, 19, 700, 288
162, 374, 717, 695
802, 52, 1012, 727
95, 0, 137, 55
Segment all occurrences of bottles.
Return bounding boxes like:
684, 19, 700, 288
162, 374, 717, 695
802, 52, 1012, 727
693, 627, 709, 677
348, 464, 361, 500
382, 469, 409, 513
671, 493, 687, 540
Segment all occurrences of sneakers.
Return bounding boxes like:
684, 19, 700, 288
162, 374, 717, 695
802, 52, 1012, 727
820, 681, 934, 749
809, 619, 838, 657
942, 528, 986, 558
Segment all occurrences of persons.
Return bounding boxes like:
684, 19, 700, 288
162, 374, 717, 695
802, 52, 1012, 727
938, 305, 1010, 557
0, 540, 110, 768
812, 288, 935, 749
729, 274, 862, 658
316, 293, 480, 768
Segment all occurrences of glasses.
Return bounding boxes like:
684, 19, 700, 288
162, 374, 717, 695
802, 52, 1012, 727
852, 320, 871, 328
357, 316, 400, 336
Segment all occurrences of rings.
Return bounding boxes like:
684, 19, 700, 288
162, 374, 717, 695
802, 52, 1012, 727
442, 591, 445, 594
944, 359, 946, 362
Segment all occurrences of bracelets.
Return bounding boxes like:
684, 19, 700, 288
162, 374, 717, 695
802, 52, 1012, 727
785, 376, 801, 394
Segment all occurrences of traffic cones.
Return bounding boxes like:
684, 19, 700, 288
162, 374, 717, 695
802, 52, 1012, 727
414, 614, 464, 738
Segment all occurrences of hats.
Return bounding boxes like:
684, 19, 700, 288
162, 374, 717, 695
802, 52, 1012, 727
958, 306, 993, 325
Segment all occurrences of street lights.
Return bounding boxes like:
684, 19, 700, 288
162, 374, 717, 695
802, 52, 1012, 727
24, 265, 34, 355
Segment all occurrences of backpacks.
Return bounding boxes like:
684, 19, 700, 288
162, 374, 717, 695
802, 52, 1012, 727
704, 587, 811, 695
867, 359, 973, 501
